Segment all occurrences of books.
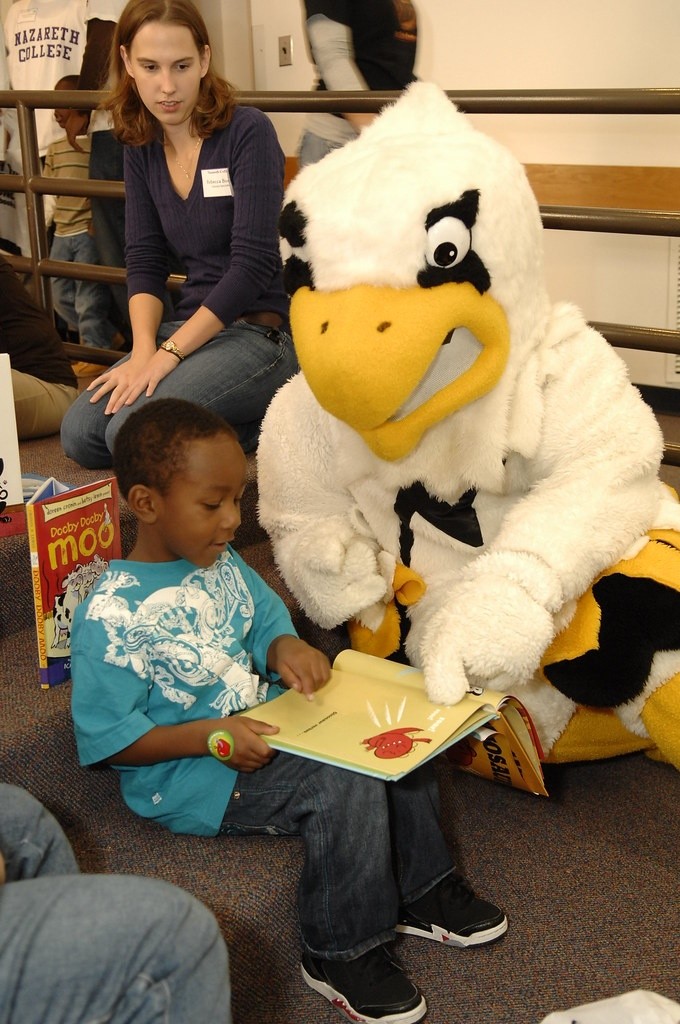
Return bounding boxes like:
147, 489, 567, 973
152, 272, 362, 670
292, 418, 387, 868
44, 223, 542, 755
236, 649, 549, 799
27, 479, 121, 690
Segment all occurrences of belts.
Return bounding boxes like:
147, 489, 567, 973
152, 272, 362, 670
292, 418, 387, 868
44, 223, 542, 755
238, 308, 281, 329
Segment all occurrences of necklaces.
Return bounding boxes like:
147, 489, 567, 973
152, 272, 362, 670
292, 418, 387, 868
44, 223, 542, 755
169, 136, 202, 178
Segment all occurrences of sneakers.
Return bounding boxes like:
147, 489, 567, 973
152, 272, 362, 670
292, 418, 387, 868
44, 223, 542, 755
393, 869, 507, 948
66, 319, 125, 377
301, 949, 426, 1024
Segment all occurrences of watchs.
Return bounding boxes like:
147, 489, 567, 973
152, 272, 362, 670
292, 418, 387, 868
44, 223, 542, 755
161, 341, 185, 360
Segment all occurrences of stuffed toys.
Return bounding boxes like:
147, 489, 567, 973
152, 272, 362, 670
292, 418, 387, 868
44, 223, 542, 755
258, 86, 680, 780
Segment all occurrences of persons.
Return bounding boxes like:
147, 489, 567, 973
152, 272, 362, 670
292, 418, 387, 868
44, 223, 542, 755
0, 783, 231, 1024
58, 0, 301, 474
68, 398, 508, 1024
0, 1, 131, 377
294, 0, 418, 173
